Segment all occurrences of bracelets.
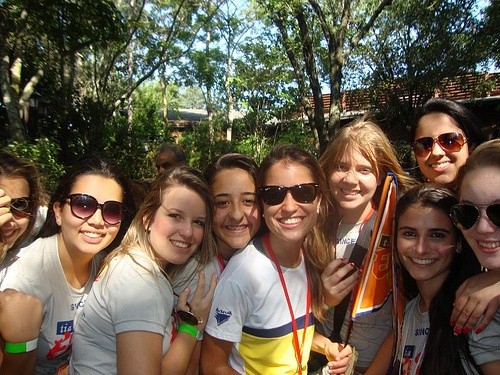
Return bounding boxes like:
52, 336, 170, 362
178, 323, 204, 341
322, 303, 332, 311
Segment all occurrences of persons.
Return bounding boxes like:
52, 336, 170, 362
0, 99, 500, 375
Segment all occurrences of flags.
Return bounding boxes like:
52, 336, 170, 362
351, 172, 407, 367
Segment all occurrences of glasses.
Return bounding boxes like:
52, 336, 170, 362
449, 203, 500, 230
173, 292, 203, 326
258, 183, 320, 205
413, 132, 468, 157
9, 197, 32, 218
66, 193, 127, 226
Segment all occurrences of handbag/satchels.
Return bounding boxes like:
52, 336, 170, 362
308, 330, 342, 375
